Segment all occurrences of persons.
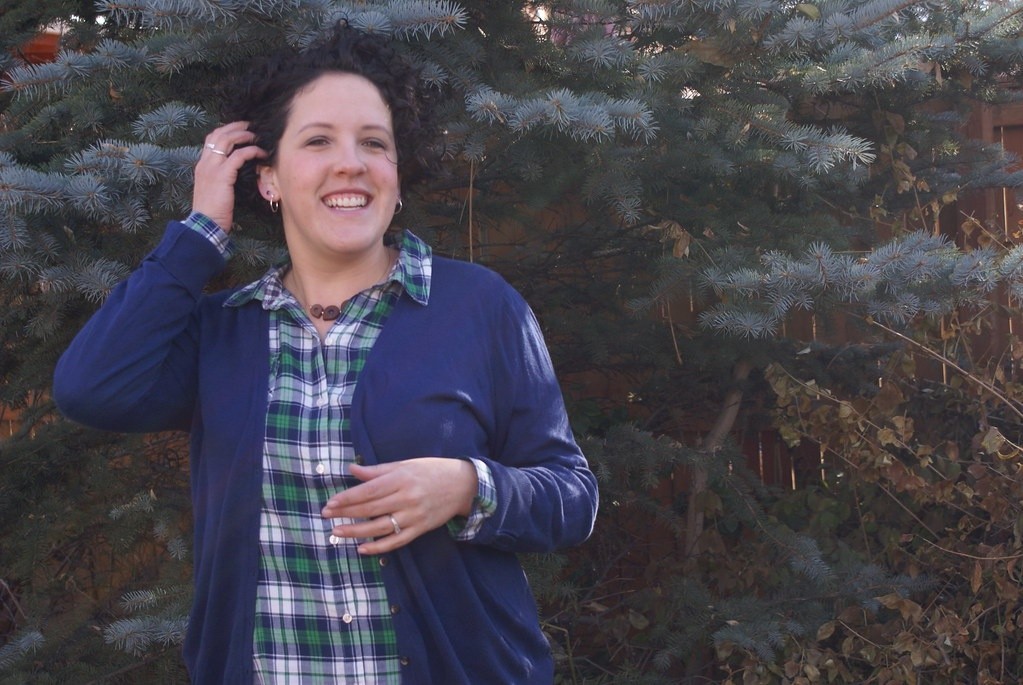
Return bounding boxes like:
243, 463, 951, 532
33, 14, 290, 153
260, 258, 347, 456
52, 19, 600, 685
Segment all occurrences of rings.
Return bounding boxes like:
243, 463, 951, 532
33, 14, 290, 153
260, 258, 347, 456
389, 515, 401, 534
211, 150, 225, 155
205, 144, 215, 149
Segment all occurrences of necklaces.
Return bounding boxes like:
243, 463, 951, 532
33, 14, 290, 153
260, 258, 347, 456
299, 300, 348, 321
